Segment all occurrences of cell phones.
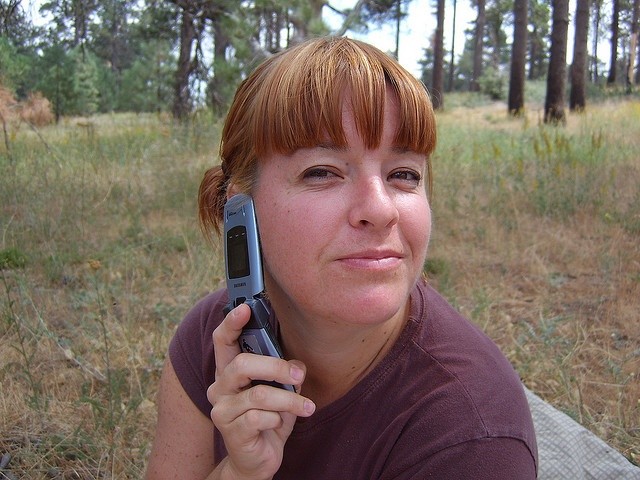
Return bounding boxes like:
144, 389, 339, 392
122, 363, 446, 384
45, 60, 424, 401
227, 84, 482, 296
223, 193, 296, 394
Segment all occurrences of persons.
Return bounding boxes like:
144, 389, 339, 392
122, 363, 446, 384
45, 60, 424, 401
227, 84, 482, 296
142, 38, 539, 479
